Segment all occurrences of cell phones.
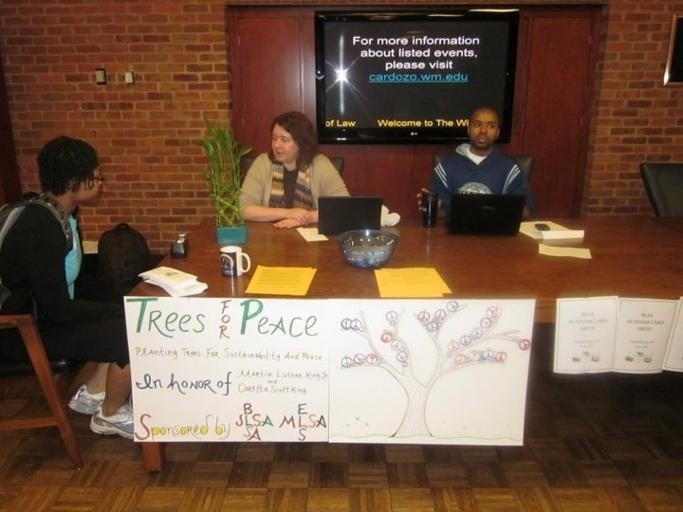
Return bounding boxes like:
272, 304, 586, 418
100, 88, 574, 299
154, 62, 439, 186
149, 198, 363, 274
535, 224, 550, 231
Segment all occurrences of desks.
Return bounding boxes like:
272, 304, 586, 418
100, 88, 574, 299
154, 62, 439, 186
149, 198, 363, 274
129, 217, 683, 471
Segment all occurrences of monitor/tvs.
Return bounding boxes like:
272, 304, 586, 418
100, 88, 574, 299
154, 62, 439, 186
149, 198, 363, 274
314, 9, 520, 144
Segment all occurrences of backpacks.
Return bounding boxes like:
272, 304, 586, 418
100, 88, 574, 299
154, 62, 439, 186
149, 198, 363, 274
98, 223, 154, 273
0, 194, 72, 252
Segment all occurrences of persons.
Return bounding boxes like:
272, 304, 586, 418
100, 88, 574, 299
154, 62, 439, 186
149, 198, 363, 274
237, 111, 350, 229
416, 106, 536, 220
0, 136, 134, 439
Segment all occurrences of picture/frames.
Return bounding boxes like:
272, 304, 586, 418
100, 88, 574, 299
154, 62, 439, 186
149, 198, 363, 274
662, 15, 683, 86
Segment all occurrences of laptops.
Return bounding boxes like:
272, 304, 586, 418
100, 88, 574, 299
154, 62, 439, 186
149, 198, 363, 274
446, 193, 528, 236
318, 196, 383, 235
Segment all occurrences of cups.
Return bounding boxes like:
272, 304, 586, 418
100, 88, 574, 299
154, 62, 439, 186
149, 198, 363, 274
167, 231, 192, 258
219, 245, 252, 277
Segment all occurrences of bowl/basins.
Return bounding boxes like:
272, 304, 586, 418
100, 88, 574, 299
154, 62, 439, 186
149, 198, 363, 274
422, 192, 439, 229
337, 229, 400, 269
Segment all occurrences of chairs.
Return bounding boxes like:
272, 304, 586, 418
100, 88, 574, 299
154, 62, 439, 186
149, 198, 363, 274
640, 163, 683, 217
0, 314, 97, 469
241, 157, 344, 187
432, 152, 534, 182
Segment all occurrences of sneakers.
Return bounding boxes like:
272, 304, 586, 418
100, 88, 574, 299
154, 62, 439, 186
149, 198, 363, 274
67, 383, 106, 415
90, 403, 134, 438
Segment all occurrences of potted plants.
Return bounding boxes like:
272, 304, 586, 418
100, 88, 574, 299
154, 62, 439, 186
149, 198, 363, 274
198, 118, 252, 245
